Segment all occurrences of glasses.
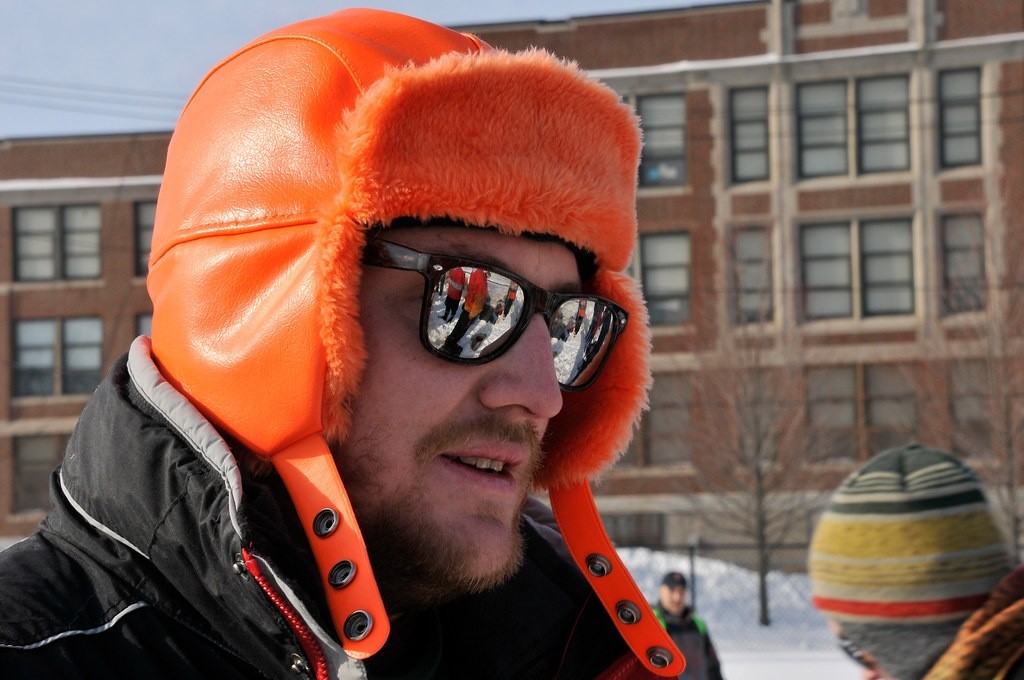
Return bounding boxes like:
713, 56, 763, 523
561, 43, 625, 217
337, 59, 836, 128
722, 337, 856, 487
365, 236, 627, 391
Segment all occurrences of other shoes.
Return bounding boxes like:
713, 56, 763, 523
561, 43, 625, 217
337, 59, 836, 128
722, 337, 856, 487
447, 319, 452, 323
438, 316, 447, 321
439, 293, 442, 296
443, 339, 464, 354
434, 290, 438, 292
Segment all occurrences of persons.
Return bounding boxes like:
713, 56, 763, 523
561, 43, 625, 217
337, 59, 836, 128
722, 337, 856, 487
649, 572, 723, 680
437, 267, 518, 351
575, 299, 603, 348
0, 7, 690, 680
806, 442, 1023, 680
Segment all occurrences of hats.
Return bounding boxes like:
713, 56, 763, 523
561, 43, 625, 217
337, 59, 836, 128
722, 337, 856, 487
146, 7, 684, 677
661, 573, 687, 589
810, 444, 1010, 625
